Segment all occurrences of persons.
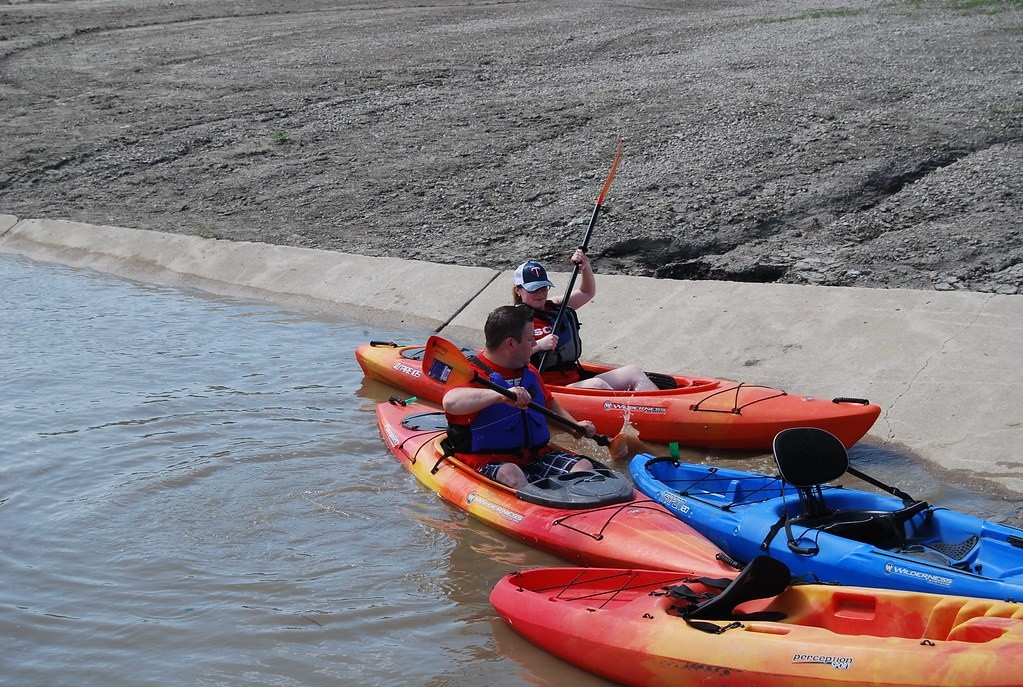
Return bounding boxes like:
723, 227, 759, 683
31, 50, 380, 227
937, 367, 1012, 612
513, 249, 661, 392
442, 305, 594, 491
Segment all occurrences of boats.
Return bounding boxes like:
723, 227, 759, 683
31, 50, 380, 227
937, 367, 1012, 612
489, 555, 1023, 687
355, 340, 882, 453
629, 427, 1023, 604
376, 396, 743, 577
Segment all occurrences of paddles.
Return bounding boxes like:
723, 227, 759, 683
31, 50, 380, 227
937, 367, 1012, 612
538, 138, 625, 374
422, 336, 658, 462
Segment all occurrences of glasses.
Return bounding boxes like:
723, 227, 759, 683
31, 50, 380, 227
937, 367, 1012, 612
521, 286, 550, 295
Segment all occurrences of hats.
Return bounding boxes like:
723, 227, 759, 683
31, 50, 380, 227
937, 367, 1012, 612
514, 260, 555, 293
